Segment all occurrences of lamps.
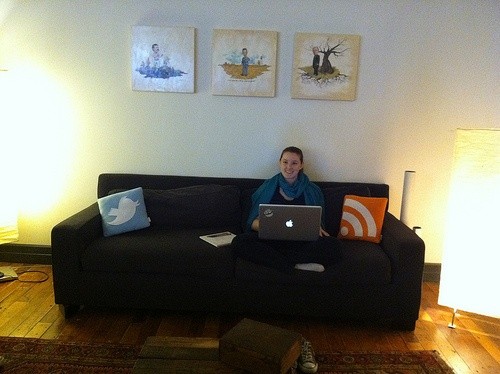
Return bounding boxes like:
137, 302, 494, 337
0, 68, 19, 283
436, 127, 500, 329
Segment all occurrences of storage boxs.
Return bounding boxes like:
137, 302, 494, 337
219, 318, 302, 374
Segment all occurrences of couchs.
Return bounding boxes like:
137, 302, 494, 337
51, 174, 425, 332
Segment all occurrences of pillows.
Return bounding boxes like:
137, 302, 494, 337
238, 185, 372, 231
106, 184, 241, 227
337, 195, 388, 244
95, 187, 151, 237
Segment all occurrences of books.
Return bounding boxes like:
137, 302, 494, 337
199, 231, 236, 248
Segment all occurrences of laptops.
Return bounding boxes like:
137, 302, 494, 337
258, 204, 322, 243
0, 266, 18, 282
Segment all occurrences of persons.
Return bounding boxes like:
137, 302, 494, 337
229, 147, 331, 272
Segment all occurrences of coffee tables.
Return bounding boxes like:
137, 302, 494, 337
131, 336, 294, 374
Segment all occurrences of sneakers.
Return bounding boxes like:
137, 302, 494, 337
299, 337, 318, 374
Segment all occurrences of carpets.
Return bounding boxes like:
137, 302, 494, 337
0, 336, 456, 374
